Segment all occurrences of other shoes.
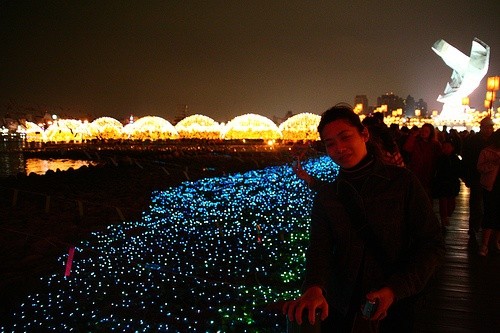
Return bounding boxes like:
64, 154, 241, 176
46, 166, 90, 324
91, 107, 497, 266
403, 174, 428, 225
495, 242, 500, 255
478, 245, 489, 256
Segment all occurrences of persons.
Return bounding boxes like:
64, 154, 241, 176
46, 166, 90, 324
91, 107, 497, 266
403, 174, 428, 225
364, 113, 500, 255
283, 103, 439, 333
295, 141, 382, 192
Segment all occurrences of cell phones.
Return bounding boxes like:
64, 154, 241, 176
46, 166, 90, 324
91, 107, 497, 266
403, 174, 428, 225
360, 299, 378, 319
294, 155, 299, 168
